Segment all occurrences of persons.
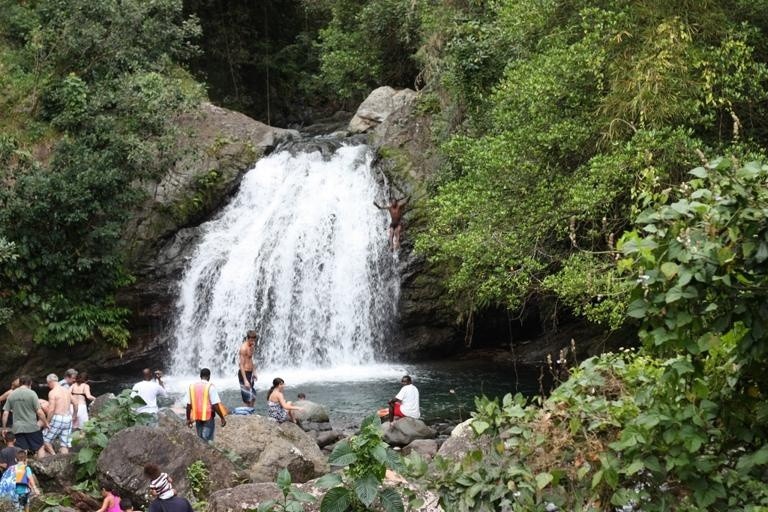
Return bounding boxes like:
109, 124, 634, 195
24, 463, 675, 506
380, 374, 421, 423
237, 330, 258, 407
297, 392, 306, 401
0, 367, 226, 512
266, 377, 304, 424
